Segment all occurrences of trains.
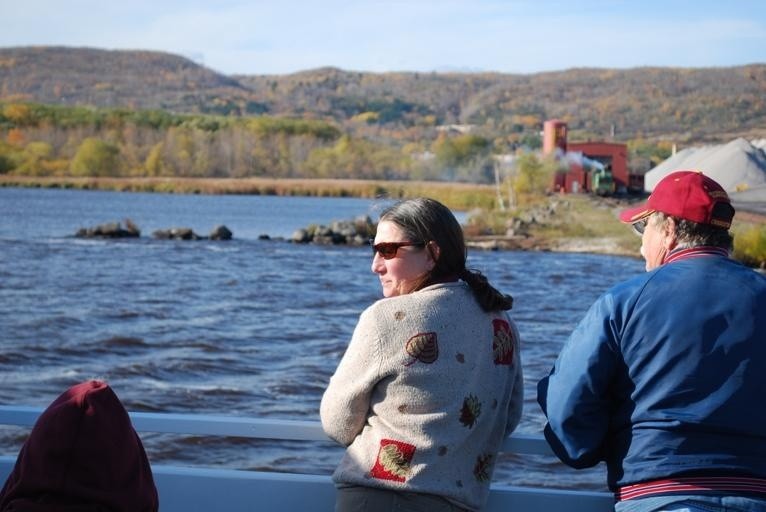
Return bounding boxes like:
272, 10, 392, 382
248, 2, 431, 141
593, 167, 613, 196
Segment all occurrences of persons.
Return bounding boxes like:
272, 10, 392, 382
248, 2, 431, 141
315, 196, 526, 510
0, 378, 160, 511
536, 171, 766, 512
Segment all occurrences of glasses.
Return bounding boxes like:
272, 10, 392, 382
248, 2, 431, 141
634, 218, 647, 234
372, 241, 424, 259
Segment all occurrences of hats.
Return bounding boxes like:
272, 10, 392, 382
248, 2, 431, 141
617, 170, 736, 229
